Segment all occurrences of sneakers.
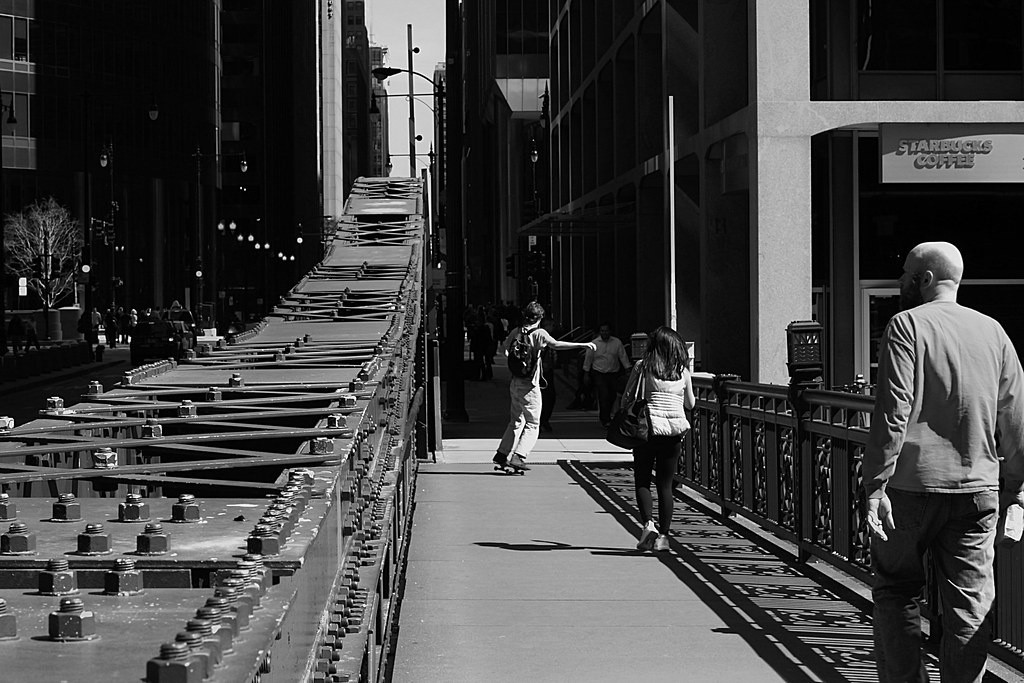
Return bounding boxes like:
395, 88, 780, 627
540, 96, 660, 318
653, 534, 669, 552
637, 521, 659, 550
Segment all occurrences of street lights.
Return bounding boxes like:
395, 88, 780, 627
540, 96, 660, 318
373, 68, 444, 345
189, 144, 248, 338
98, 131, 119, 323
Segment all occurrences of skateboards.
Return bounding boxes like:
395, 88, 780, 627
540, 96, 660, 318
492, 460, 532, 476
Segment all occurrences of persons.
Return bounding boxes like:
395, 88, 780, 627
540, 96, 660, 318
9, 312, 41, 354
582, 323, 632, 429
539, 316, 557, 435
81, 305, 166, 349
865, 240, 1023, 683
621, 326, 695, 552
493, 301, 598, 470
464, 298, 523, 378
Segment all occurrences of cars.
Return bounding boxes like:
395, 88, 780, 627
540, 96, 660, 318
130, 318, 194, 367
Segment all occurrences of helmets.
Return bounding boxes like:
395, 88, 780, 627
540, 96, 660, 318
523, 301, 544, 319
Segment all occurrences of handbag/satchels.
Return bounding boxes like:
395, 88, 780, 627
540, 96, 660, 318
606, 360, 648, 449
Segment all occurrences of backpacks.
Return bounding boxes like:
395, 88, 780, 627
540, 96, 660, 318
508, 325, 539, 378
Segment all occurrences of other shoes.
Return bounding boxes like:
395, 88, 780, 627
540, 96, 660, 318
602, 421, 610, 430
493, 453, 510, 466
540, 417, 552, 432
511, 454, 530, 470
